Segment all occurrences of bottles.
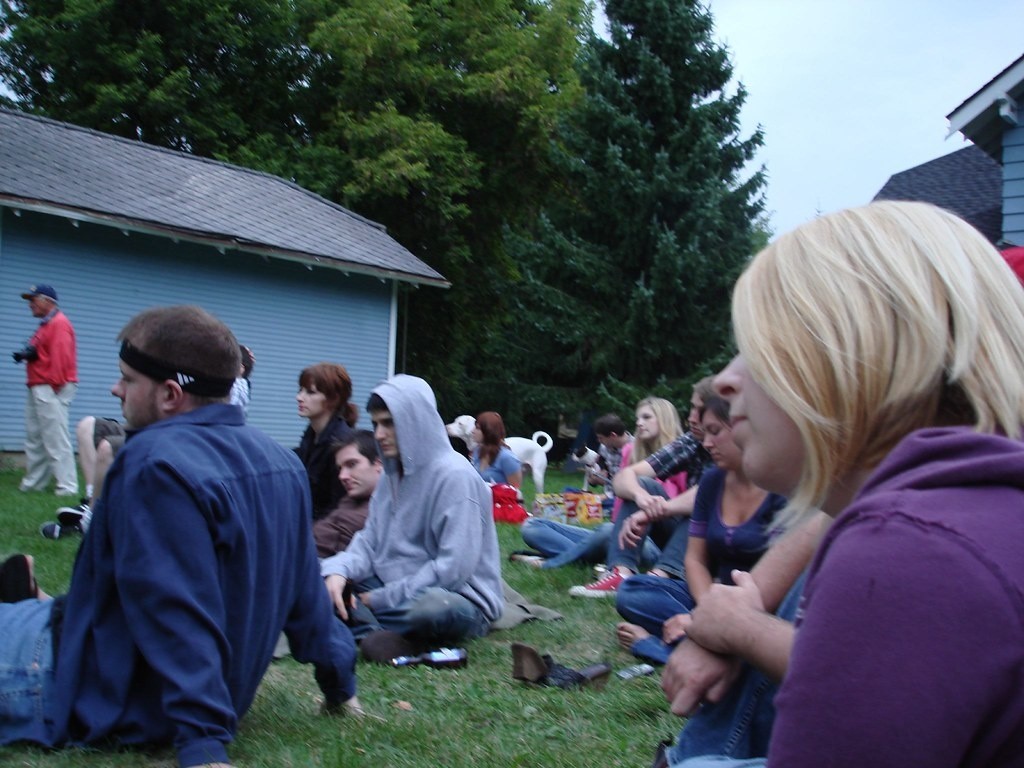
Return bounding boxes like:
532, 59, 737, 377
392, 645, 467, 669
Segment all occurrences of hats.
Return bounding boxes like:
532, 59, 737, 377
19, 283, 59, 304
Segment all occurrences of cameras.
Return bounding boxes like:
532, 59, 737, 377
12, 346, 37, 362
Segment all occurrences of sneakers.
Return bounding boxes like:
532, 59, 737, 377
566, 568, 627, 599
55, 500, 89, 525
38, 519, 91, 540
508, 551, 545, 567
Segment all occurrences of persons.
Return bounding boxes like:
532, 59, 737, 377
565, 413, 636, 516
40, 342, 256, 540
311, 429, 383, 560
570, 376, 721, 600
656, 507, 837, 768
289, 362, 358, 524
468, 410, 523, 488
712, 199, 1024, 767
0, 302, 367, 768
508, 396, 688, 570
615, 394, 789, 667
18, 285, 80, 495
319, 372, 504, 662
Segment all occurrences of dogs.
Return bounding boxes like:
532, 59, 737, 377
571, 442, 614, 492
445, 415, 553, 496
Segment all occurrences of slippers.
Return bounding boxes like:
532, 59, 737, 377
527, 650, 610, 693
